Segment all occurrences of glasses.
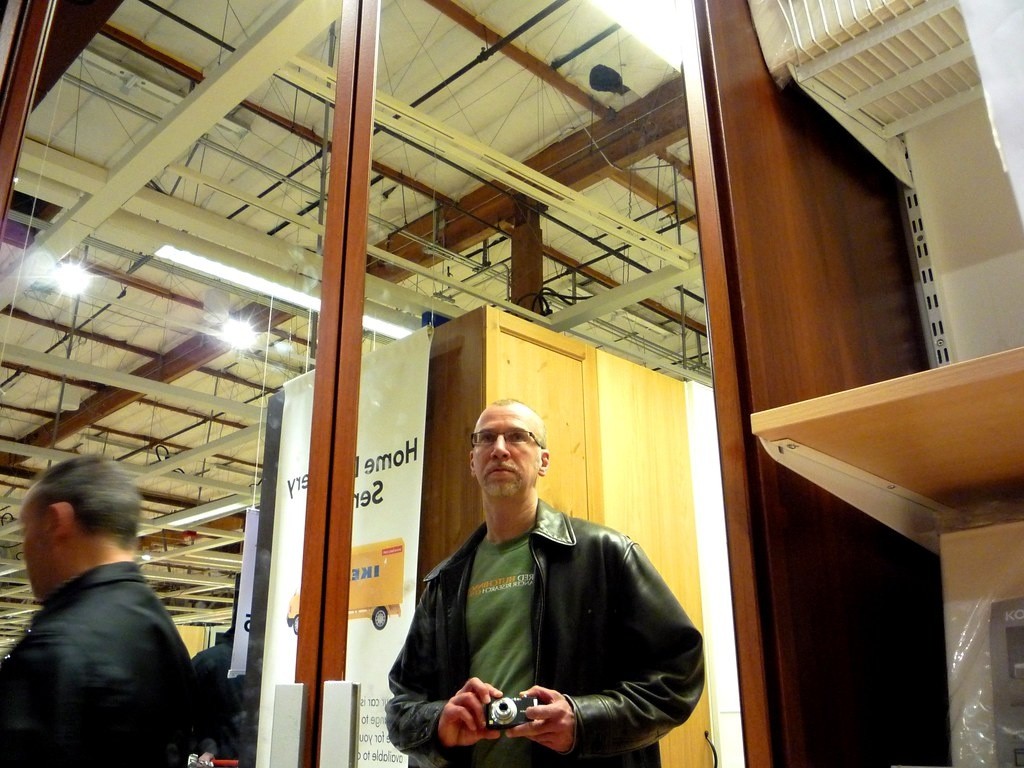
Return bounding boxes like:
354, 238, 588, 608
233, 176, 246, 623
471, 430, 545, 450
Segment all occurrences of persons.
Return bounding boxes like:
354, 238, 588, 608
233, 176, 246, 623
384, 396, 705, 768
189, 623, 250, 768
0, 454, 196, 768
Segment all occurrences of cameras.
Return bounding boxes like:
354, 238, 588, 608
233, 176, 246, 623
486, 697, 539, 729
